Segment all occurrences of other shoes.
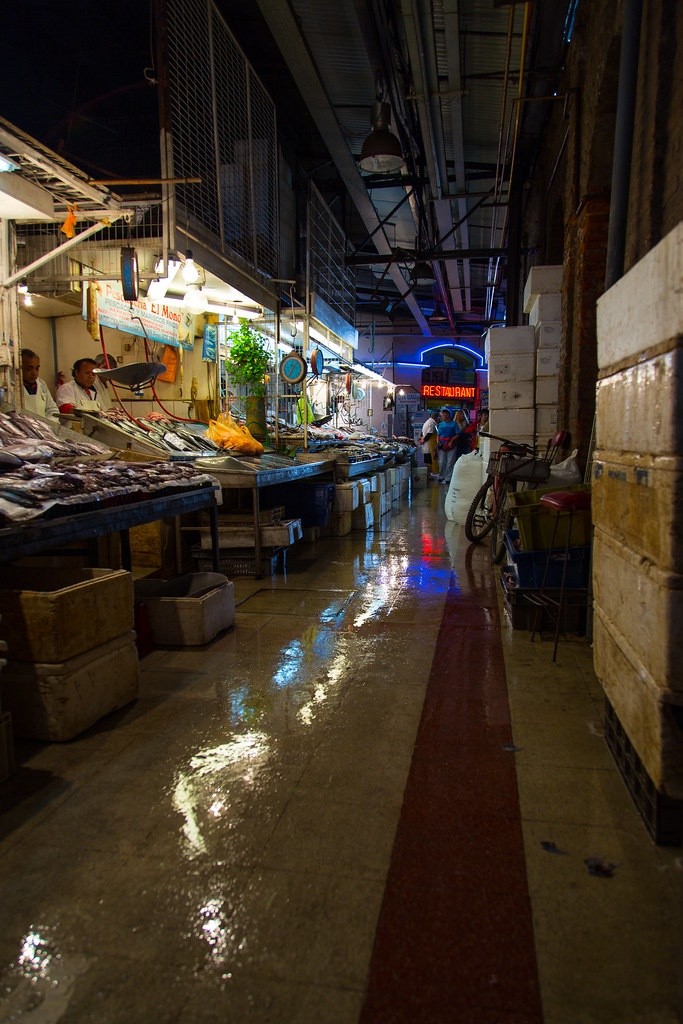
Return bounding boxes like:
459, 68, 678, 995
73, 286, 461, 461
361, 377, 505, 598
427, 472, 437, 480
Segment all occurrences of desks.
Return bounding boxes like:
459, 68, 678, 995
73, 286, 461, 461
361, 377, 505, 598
175, 458, 335, 580
0, 486, 220, 574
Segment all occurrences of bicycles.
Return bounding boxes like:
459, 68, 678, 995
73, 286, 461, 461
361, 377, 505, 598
464, 430, 535, 566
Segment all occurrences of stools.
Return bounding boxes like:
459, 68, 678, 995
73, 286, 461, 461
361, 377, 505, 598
523, 489, 592, 662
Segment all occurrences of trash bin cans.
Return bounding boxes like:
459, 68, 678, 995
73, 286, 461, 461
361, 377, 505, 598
299, 482, 332, 527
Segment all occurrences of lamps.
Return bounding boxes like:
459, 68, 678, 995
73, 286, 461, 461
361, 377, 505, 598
375, 296, 392, 313
358, 101, 403, 172
408, 263, 436, 286
429, 305, 447, 321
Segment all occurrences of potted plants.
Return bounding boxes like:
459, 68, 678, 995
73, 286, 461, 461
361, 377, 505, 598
220, 325, 271, 435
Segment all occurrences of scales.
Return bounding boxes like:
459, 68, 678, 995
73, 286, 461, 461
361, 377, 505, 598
91, 247, 166, 391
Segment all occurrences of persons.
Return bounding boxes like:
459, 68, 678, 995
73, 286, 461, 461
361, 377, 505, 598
9, 348, 60, 423
420, 409, 489, 484
56, 358, 106, 414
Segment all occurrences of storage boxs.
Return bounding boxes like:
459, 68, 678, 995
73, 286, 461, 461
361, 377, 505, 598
216, 136, 291, 271
475, 219, 683, 844
137, 582, 234, 647
189, 462, 427, 575
0, 563, 140, 785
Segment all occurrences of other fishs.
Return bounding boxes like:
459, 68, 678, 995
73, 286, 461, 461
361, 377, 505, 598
1, 410, 204, 518
102, 406, 216, 457
